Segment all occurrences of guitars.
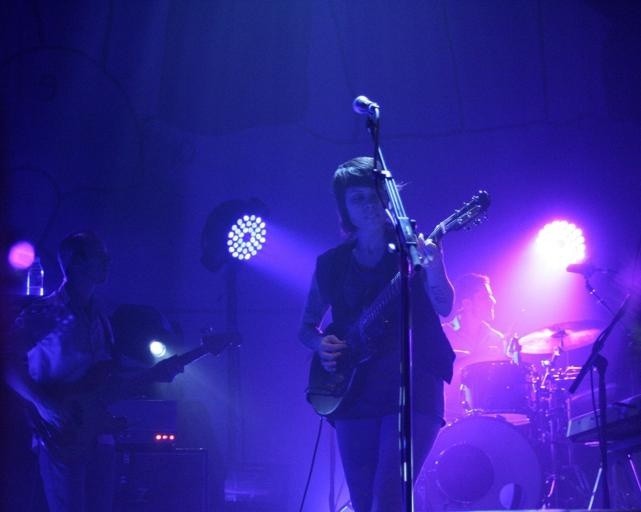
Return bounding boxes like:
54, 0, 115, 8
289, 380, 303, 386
309, 189, 491, 417
25, 327, 227, 470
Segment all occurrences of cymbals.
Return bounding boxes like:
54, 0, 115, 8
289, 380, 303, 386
518, 321, 603, 355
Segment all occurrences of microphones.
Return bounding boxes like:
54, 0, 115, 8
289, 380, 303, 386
566, 263, 596, 276
352, 95, 380, 115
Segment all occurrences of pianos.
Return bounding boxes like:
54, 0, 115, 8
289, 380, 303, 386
566, 394, 641, 443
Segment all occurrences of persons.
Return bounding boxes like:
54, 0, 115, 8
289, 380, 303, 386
0, 232, 184, 512
298, 156, 455, 512
442, 273, 512, 426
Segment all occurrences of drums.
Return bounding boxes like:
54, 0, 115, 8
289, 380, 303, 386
412, 410, 543, 510
461, 360, 534, 426
